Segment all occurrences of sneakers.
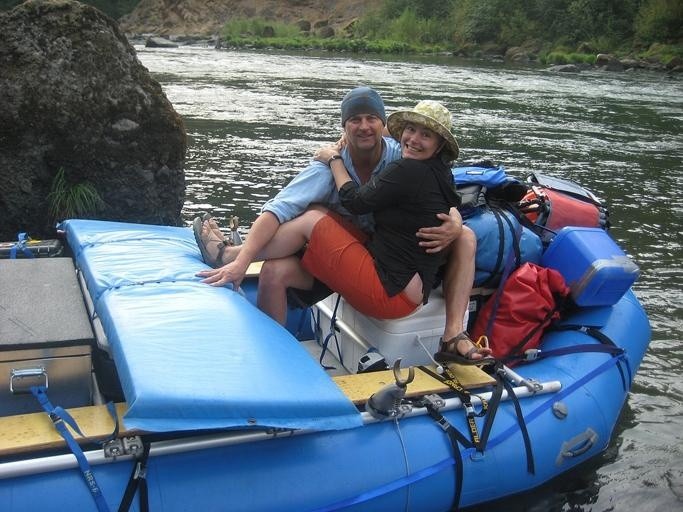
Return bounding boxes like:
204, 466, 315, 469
192, 215, 228, 269
202, 212, 234, 250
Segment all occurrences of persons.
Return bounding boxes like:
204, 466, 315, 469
194, 86, 498, 366
192, 100, 462, 318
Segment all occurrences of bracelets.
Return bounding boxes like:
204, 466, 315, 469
327, 155, 342, 168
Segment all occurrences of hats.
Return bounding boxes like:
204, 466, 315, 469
339, 85, 386, 129
385, 98, 461, 163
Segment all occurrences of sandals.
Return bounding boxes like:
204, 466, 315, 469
431, 331, 496, 366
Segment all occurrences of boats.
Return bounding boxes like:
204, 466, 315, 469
0, 220, 652, 512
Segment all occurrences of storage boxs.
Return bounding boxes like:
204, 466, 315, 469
0, 256, 97, 417
542, 224, 639, 309
310, 289, 472, 373
